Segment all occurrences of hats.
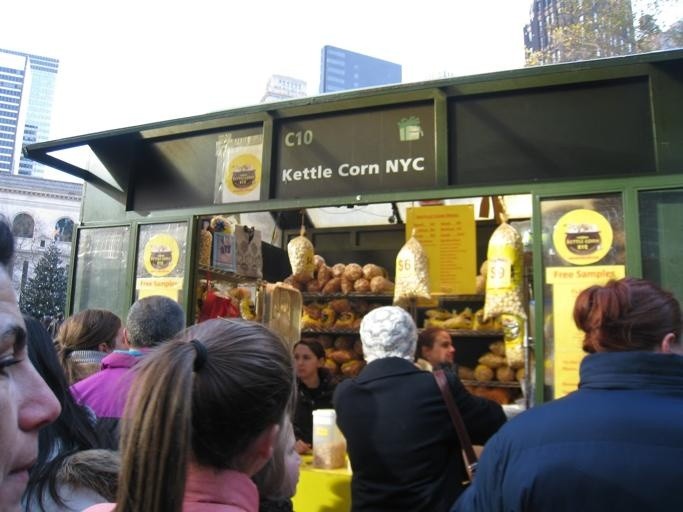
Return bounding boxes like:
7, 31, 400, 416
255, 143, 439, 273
359, 305, 418, 365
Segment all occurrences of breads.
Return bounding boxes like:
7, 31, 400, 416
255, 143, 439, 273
316, 334, 365, 375
285, 257, 394, 294
457, 340, 517, 383
476, 260, 486, 294
424, 307, 501, 334
299, 298, 379, 332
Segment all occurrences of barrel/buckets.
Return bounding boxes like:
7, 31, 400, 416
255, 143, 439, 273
311, 408, 346, 469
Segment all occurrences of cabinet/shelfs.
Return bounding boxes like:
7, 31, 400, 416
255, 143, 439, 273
295, 266, 533, 408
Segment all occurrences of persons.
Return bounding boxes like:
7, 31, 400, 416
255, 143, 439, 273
83, 317, 297, 512
416, 326, 457, 372
65, 295, 186, 453
332, 306, 508, 512
22, 316, 109, 512
450, 275, 683, 511
56, 308, 126, 386
251, 415, 302, 512
291, 337, 342, 454
1, 219, 62, 512
